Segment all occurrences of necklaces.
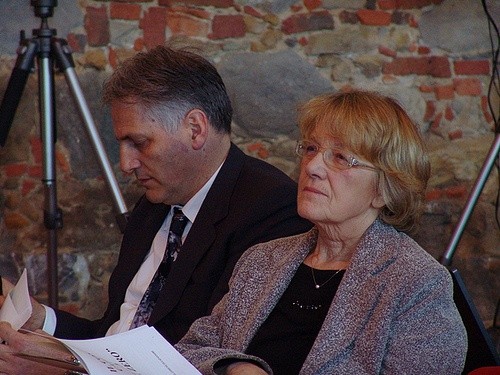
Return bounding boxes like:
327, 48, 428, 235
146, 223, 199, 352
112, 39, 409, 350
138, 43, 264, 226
309, 253, 348, 288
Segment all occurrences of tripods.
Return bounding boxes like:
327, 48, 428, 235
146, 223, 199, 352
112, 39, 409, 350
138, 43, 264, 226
0, 0, 130, 309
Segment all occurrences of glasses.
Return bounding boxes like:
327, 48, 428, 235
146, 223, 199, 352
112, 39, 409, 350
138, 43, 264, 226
296, 137, 379, 172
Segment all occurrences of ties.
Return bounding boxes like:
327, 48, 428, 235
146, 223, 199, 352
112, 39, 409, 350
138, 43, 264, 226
128, 207, 189, 331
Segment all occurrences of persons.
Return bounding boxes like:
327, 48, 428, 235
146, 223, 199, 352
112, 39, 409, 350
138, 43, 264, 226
173, 88, 468, 375
0, 44, 316, 346
